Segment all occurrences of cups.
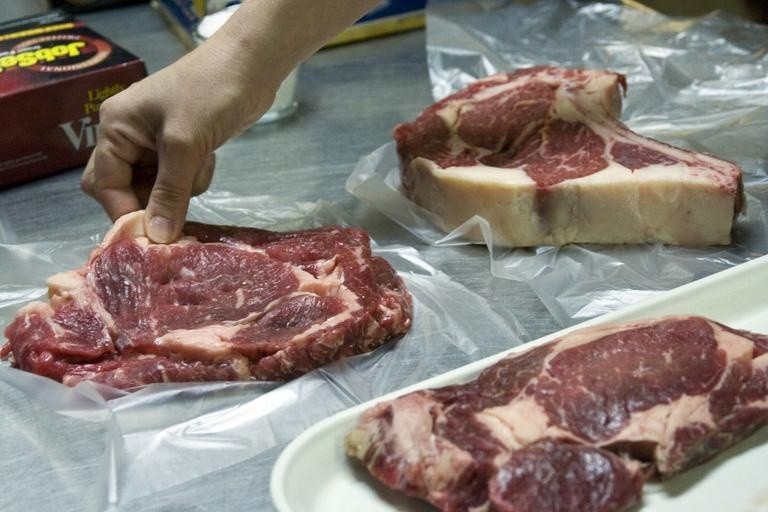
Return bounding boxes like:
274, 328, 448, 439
191, 11, 303, 123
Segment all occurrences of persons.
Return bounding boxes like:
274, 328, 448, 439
79, 0, 381, 245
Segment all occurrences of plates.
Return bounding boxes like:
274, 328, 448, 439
269, 253, 768, 511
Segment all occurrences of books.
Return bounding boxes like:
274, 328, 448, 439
151, 0, 536, 54
